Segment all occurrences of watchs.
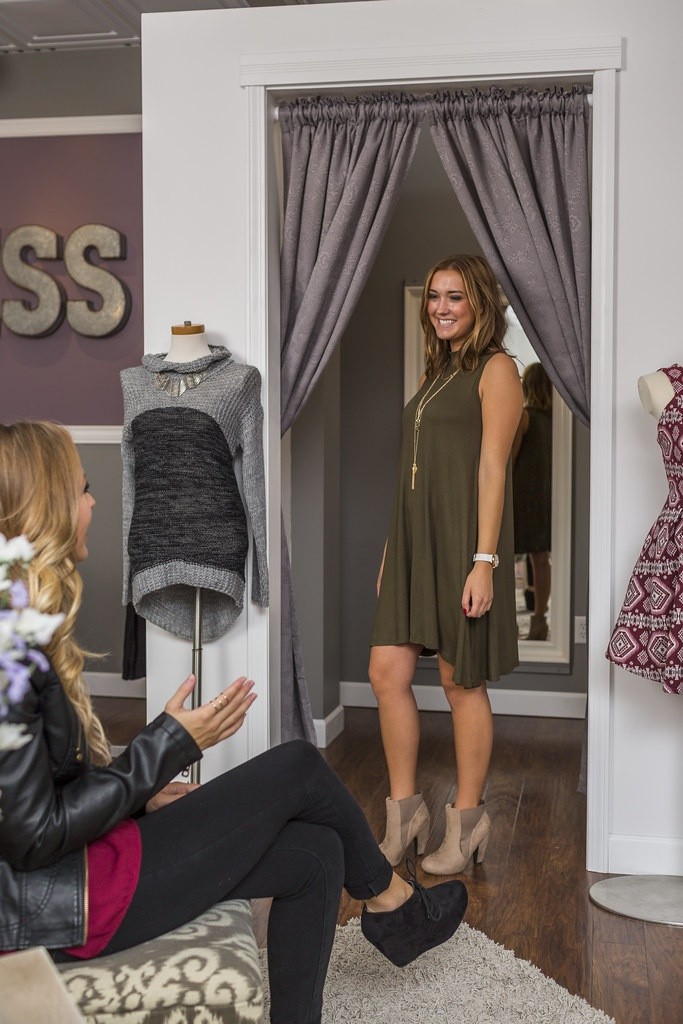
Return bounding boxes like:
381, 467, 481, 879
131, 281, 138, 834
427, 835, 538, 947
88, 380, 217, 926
472, 553, 499, 569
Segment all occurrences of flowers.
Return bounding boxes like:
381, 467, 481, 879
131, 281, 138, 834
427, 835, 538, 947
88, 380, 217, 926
0, 532, 67, 752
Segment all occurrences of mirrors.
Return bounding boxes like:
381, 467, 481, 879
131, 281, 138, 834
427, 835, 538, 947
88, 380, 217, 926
404, 284, 573, 666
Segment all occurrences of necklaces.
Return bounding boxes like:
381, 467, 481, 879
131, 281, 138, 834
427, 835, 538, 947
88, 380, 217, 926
410, 359, 462, 491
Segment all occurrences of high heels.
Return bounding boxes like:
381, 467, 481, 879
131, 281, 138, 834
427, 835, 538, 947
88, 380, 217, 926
359, 855, 467, 967
525, 615, 548, 640
422, 799, 491, 874
524, 589, 549, 612
380, 790, 432, 866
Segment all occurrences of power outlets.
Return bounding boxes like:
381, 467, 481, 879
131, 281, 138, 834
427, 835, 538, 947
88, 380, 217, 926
574, 615, 587, 644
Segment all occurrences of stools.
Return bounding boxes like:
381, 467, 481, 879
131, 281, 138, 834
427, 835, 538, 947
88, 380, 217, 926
55, 899, 265, 1024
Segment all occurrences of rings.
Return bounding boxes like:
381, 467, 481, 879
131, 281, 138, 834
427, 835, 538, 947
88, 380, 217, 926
210, 693, 229, 712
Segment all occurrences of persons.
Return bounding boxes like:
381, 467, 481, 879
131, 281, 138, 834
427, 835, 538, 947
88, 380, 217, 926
513, 363, 552, 640
605, 361, 683, 694
366, 255, 523, 878
118, 320, 269, 643
0, 421, 467, 1024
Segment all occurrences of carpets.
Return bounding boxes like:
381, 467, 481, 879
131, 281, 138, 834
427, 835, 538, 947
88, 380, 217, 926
257, 917, 617, 1024
